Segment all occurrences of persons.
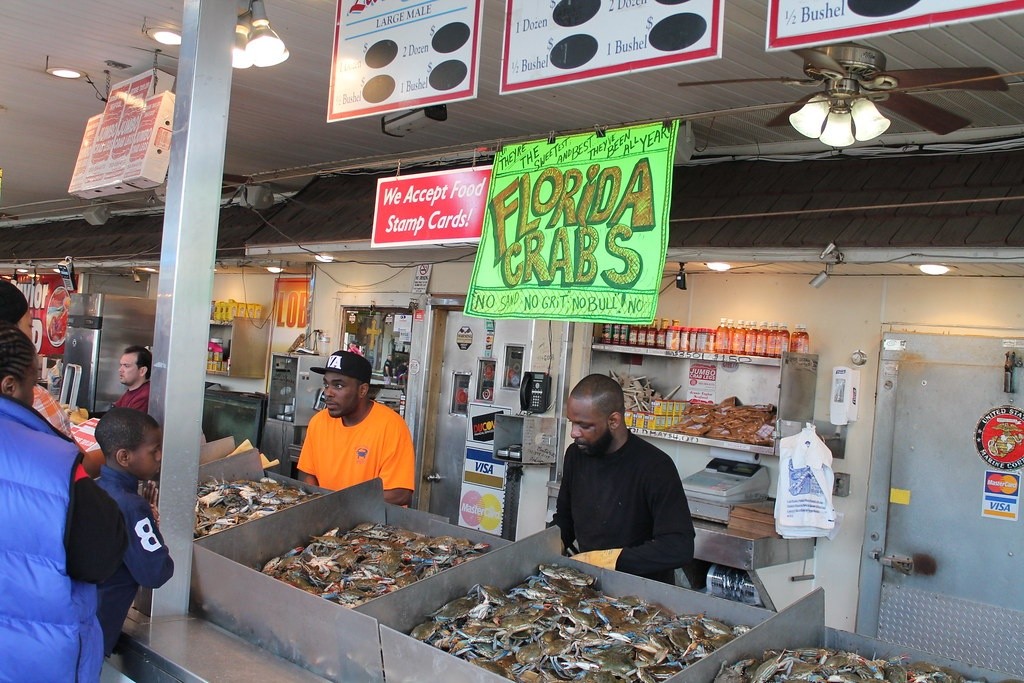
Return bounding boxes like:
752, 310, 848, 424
548, 374, 696, 585
95, 408, 175, 657
296, 350, 414, 507
113, 346, 152, 413
0, 321, 127, 683
0, 279, 107, 479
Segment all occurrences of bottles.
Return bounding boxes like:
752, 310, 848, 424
601, 315, 810, 362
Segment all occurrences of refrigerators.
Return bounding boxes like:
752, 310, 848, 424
58, 293, 156, 414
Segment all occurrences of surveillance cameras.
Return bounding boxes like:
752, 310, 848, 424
676, 271, 687, 290
809, 271, 829, 289
134, 273, 142, 282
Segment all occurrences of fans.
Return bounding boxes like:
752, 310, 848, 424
675, 43, 1011, 137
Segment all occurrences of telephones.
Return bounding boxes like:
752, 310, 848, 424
520, 371, 552, 413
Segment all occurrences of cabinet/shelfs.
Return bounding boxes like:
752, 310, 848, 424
204, 316, 270, 380
583, 343, 781, 455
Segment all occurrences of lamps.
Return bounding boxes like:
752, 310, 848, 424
788, 85, 892, 147
232, 0, 290, 68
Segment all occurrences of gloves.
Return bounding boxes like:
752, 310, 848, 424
571, 547, 621, 570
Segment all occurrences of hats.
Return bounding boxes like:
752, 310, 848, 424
310, 352, 372, 383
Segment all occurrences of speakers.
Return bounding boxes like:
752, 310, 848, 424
83, 206, 110, 225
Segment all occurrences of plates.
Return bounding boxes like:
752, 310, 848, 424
46, 285, 69, 347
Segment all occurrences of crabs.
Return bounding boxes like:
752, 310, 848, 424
199, 521, 490, 678
193, 475, 322, 538
410, 563, 750, 683
713, 648, 989, 683
